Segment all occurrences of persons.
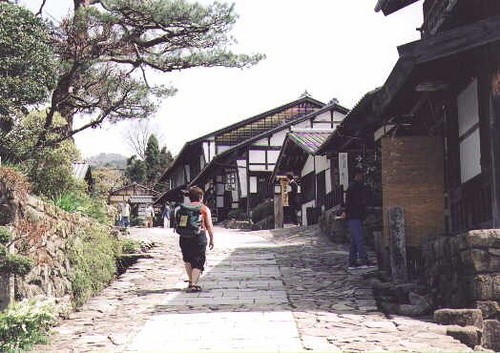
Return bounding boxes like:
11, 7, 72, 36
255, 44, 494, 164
144, 203, 155, 229
120, 199, 132, 229
174, 185, 214, 292
345, 167, 374, 270
286, 171, 301, 228
162, 201, 171, 229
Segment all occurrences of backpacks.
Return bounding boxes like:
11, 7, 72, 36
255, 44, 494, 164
175, 204, 204, 235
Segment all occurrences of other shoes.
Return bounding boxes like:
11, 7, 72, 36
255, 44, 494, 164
348, 262, 376, 268
187, 283, 202, 293
